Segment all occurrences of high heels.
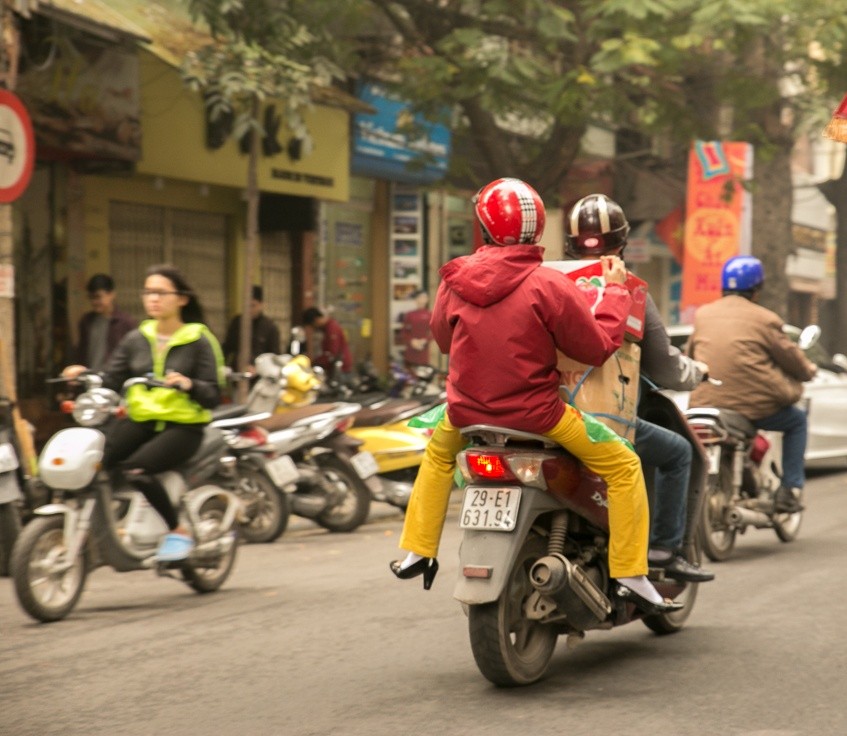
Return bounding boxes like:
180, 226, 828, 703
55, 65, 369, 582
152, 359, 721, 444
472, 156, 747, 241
390, 557, 439, 590
608, 576, 685, 615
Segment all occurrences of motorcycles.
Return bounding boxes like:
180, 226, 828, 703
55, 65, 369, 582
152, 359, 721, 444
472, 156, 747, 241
449, 354, 722, 688
684, 321, 820, 564
6, 324, 451, 624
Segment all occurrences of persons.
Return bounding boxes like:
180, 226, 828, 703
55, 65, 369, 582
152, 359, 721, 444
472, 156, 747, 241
75, 274, 135, 368
562, 192, 713, 581
686, 253, 818, 513
390, 175, 685, 613
398, 290, 434, 379
224, 284, 280, 402
305, 306, 353, 378
60, 265, 225, 564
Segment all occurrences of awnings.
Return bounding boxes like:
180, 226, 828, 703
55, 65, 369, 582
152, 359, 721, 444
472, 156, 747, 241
36, 0, 381, 117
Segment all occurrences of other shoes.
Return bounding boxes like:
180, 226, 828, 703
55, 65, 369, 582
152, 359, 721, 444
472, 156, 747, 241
775, 487, 805, 512
648, 552, 715, 582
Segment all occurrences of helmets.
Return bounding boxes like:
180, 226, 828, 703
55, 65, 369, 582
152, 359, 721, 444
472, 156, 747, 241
471, 177, 547, 246
721, 256, 765, 290
566, 193, 630, 252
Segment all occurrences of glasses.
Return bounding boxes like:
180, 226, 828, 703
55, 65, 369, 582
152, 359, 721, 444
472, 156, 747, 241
138, 288, 190, 297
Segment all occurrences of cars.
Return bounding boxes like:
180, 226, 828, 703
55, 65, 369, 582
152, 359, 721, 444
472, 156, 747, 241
665, 323, 847, 476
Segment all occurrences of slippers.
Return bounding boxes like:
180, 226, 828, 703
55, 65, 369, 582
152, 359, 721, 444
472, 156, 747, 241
156, 534, 194, 560
